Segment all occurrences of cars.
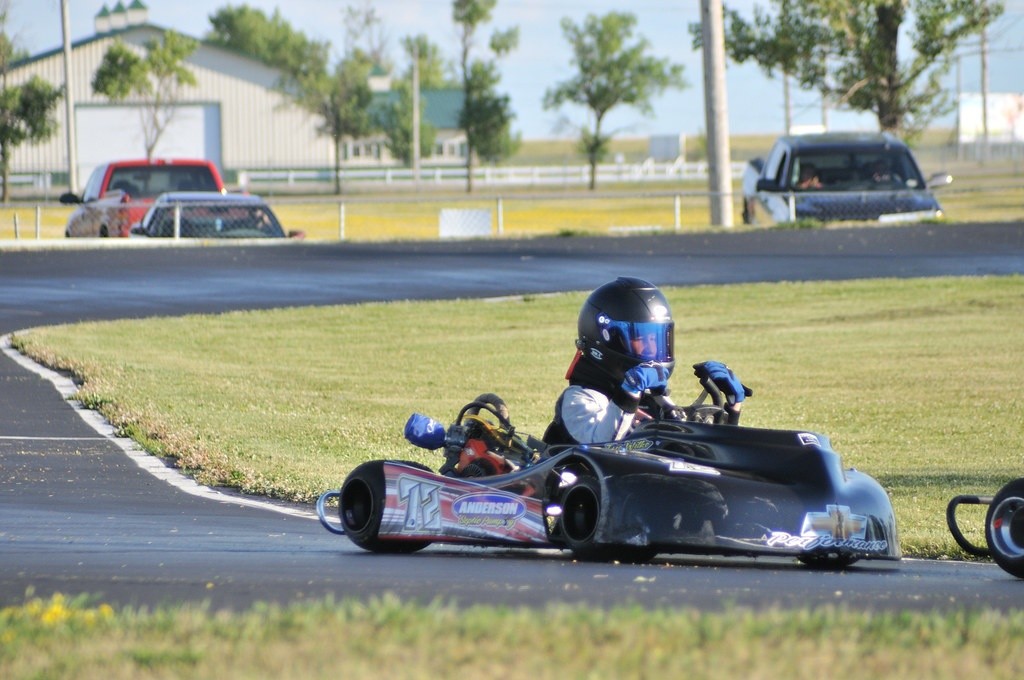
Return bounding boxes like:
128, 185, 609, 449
129, 191, 307, 239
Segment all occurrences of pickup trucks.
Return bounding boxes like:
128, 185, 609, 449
59, 158, 226, 237
742, 132, 952, 229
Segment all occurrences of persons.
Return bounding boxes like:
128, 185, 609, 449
543, 276, 744, 454
797, 165, 822, 189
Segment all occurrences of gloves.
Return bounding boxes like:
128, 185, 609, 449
621, 360, 670, 399
693, 360, 745, 407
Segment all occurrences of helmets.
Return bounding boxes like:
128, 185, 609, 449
575, 275, 676, 393
